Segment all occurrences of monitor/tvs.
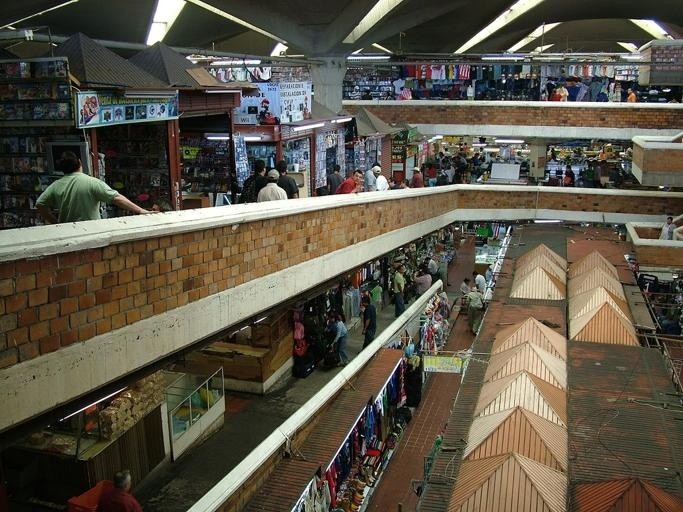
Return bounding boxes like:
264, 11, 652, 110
45, 142, 93, 175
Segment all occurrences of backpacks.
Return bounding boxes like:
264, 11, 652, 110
293, 294, 341, 378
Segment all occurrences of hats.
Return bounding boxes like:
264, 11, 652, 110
268, 169, 280, 180
372, 166, 381, 174
410, 167, 420, 172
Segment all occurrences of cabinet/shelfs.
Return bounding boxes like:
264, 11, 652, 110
342, 65, 398, 100
178, 127, 232, 208
0, 57, 98, 230
99, 119, 181, 218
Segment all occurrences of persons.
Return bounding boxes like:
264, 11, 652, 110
324, 308, 350, 366
545, 148, 632, 189
627, 88, 637, 102
35, 149, 160, 225
244, 159, 299, 202
668, 97, 679, 103
596, 88, 609, 101
393, 257, 493, 335
384, 91, 391, 100
362, 295, 376, 350
540, 82, 570, 101
350, 85, 373, 99
95, 470, 142, 512
659, 216, 676, 240
326, 146, 493, 195
